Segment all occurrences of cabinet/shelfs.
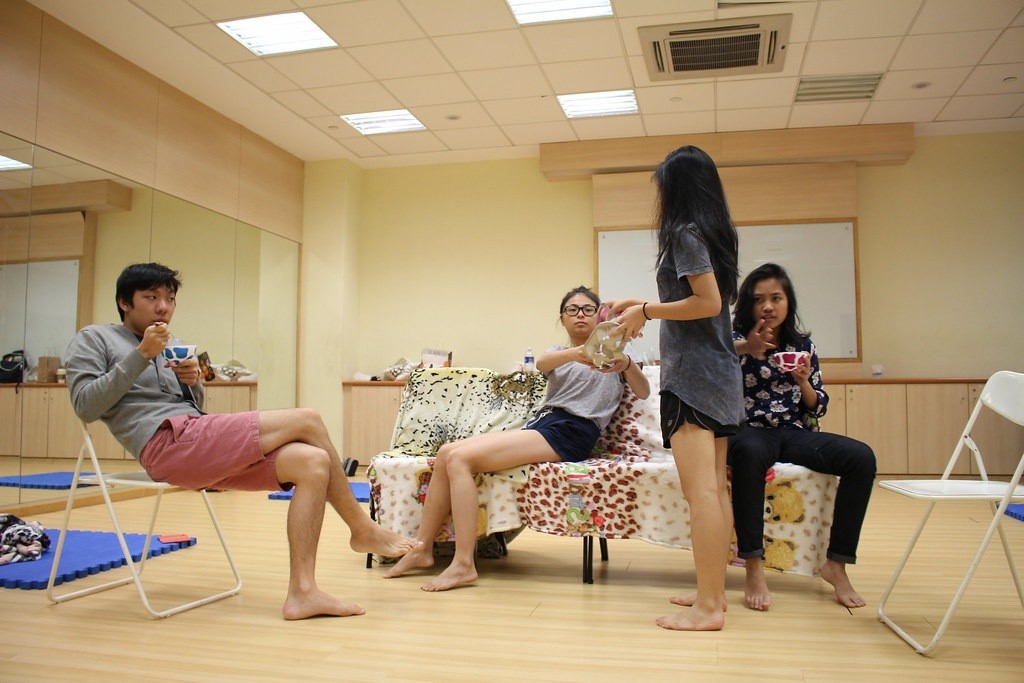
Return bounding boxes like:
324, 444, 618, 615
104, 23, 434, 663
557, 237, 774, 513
1, 380, 258, 461
342, 378, 403, 465
820, 376, 1024, 475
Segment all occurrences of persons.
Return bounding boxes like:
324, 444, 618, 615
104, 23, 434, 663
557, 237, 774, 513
601, 145, 744, 631
64, 263, 424, 620
726, 262, 877, 611
383, 286, 650, 592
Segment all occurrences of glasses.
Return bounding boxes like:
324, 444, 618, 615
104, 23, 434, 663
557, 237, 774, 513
561, 303, 597, 317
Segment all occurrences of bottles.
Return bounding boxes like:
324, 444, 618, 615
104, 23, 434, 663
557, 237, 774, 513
523, 347, 534, 372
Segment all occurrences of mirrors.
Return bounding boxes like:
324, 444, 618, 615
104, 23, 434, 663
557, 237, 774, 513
0, 132, 300, 512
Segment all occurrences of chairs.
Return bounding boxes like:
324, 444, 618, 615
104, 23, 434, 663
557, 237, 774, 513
45, 420, 244, 618
878, 369, 1024, 655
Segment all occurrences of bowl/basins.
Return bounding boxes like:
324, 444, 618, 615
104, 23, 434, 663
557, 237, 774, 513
775, 351, 811, 371
161, 345, 197, 366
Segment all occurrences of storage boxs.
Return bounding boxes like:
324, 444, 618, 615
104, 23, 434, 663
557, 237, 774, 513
419, 348, 452, 368
37, 356, 61, 382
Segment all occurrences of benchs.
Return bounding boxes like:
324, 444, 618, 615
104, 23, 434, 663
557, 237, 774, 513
366, 369, 842, 586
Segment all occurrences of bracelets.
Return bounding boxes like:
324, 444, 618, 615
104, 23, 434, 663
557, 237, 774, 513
625, 354, 631, 370
643, 302, 651, 321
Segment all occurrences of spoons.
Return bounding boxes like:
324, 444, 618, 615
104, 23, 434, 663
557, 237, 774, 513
152, 322, 183, 345
